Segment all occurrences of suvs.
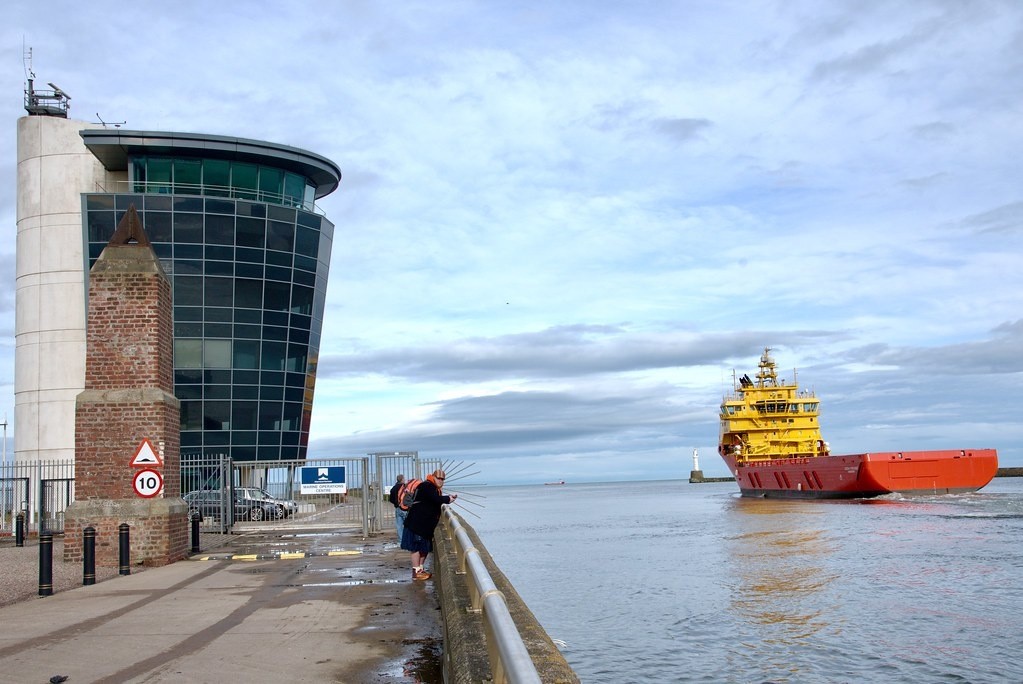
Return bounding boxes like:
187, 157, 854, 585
219, 487, 299, 520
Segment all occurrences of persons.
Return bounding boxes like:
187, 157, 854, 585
400, 470, 456, 580
389, 475, 409, 547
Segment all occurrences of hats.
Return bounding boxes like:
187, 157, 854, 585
435, 470, 445, 480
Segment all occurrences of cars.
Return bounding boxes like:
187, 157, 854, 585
182, 490, 277, 521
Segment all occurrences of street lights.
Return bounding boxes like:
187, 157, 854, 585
0, 413, 8, 530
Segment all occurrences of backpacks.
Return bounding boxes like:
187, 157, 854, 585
398, 478, 423, 511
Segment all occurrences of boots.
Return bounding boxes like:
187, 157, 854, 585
419, 565, 432, 578
412, 567, 429, 581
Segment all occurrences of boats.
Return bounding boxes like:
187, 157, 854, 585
718, 345, 998, 500
544, 480, 564, 485
